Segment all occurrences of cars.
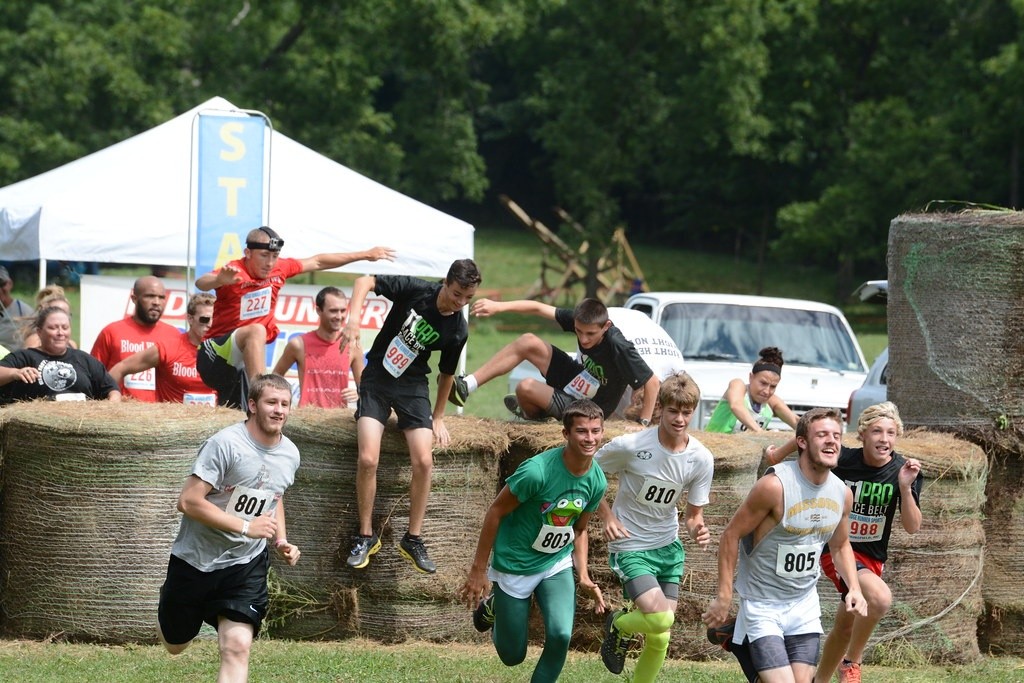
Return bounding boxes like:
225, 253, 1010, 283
846, 347, 889, 432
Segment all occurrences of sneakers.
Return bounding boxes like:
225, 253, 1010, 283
473, 593, 495, 633
436, 373, 469, 406
707, 616, 738, 651
838, 654, 861, 683
504, 393, 549, 421
348, 531, 382, 569
601, 608, 636, 674
397, 530, 436, 574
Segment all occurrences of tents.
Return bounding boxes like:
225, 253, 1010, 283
0, 94, 476, 418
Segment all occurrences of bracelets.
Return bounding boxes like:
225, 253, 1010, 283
274, 539, 286, 544
240, 520, 248, 535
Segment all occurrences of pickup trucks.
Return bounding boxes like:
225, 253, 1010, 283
507, 291, 869, 435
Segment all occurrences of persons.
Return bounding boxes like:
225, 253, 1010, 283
195, 227, 398, 409
87, 275, 181, 403
333, 258, 482, 575
155, 374, 300, 683
702, 407, 870, 683
832, 402, 921, 683
591, 371, 715, 683
107, 294, 221, 406
457, 398, 606, 683
435, 297, 660, 428
706, 345, 808, 433
0, 263, 123, 402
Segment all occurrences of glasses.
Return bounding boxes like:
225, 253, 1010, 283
0, 278, 9, 289
194, 315, 214, 323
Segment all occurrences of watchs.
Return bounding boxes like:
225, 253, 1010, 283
637, 418, 653, 428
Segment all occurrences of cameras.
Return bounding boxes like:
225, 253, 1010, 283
269, 238, 284, 250
